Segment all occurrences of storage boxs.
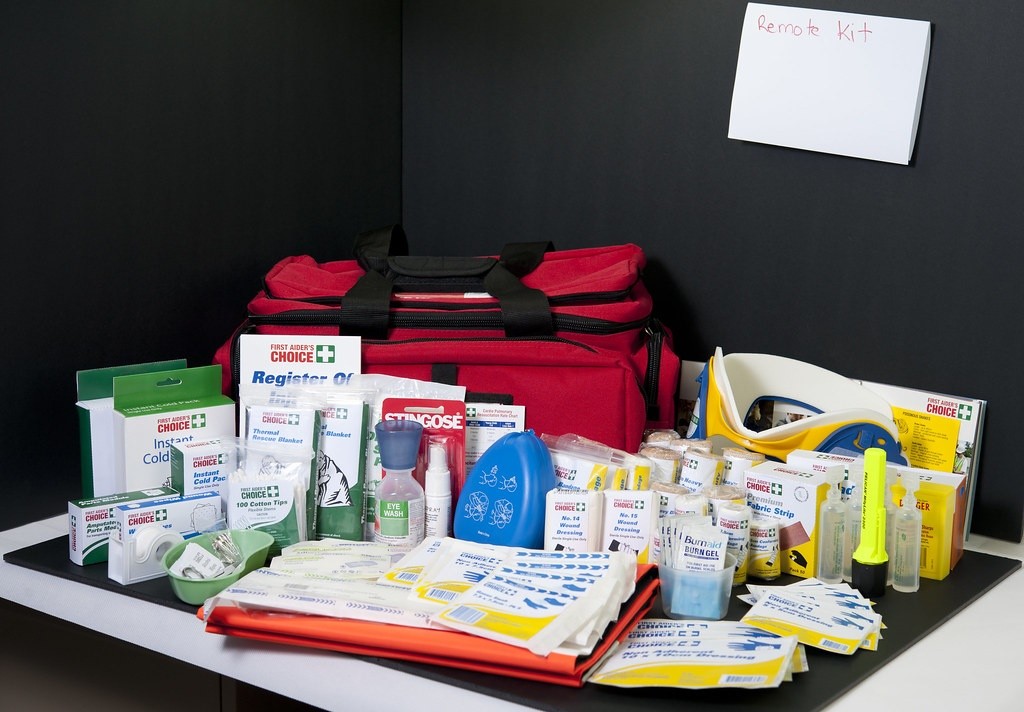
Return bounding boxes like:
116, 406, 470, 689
747, 461, 828, 579
75, 360, 188, 493
237, 330, 363, 540
246, 406, 316, 538
658, 558, 738, 619
108, 492, 221, 590
105, 366, 235, 501
767, 378, 986, 543
64, 489, 178, 568
172, 439, 232, 499
787, 448, 967, 581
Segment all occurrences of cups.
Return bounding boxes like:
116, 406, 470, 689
375, 420, 423, 471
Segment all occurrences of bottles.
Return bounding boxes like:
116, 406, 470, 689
374, 467, 425, 548
425, 444, 452, 538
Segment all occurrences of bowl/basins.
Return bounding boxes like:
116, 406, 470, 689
160, 528, 274, 606
656, 549, 737, 622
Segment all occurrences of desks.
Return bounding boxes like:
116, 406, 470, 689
0, 508, 1024, 712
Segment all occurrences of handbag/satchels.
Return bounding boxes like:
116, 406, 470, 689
213, 240, 681, 455
